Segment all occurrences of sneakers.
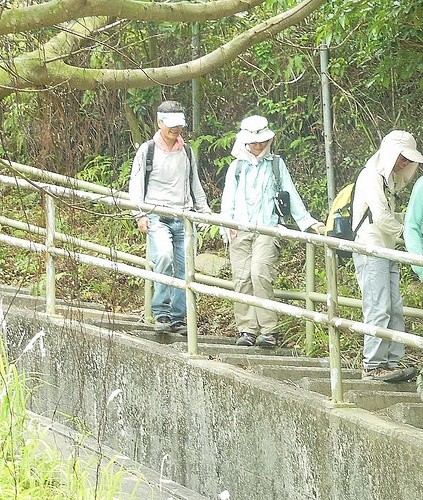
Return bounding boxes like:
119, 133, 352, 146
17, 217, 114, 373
362, 368, 402, 381
154, 317, 171, 333
169, 323, 188, 335
256, 333, 278, 346
388, 368, 417, 381
236, 333, 256, 346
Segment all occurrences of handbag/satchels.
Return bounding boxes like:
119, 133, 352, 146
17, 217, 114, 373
271, 155, 290, 217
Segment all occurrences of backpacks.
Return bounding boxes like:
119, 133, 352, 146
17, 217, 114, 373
325, 167, 372, 257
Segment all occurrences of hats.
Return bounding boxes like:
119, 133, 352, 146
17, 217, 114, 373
231, 115, 275, 165
365, 130, 423, 191
157, 112, 186, 127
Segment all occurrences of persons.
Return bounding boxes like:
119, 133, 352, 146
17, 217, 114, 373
403, 176, 423, 278
352, 130, 423, 382
129, 101, 211, 335
220, 115, 324, 349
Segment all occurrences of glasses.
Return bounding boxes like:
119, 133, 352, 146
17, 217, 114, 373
399, 154, 413, 163
